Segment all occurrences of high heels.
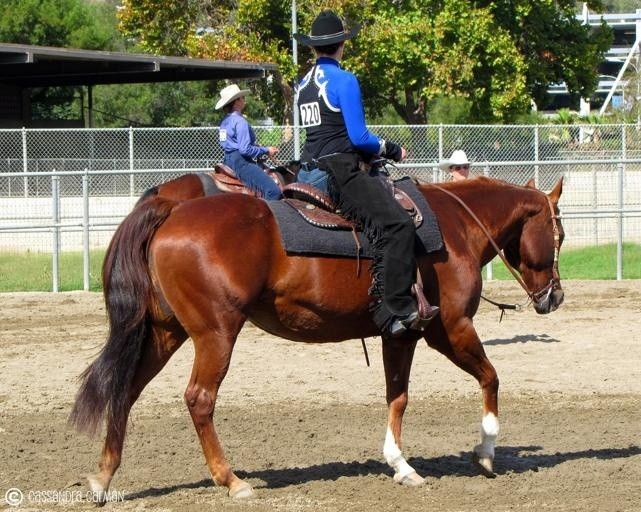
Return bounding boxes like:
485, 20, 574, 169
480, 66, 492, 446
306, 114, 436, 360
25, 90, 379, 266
391, 306, 439, 334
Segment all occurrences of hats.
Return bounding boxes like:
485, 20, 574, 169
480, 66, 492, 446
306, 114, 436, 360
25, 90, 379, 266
293, 9, 360, 46
215, 83, 250, 110
439, 149, 472, 171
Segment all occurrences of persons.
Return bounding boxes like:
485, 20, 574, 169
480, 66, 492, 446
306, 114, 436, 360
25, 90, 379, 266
293, 11, 440, 335
439, 150, 472, 183
215, 84, 283, 201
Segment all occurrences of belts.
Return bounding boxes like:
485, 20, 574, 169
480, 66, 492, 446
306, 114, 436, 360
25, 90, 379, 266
300, 157, 319, 172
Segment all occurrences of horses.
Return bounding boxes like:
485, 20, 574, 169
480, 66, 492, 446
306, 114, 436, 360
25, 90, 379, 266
133, 161, 303, 205
63, 175, 566, 507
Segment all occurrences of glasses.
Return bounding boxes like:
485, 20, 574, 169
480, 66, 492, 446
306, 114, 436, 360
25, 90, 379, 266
454, 166, 468, 170
